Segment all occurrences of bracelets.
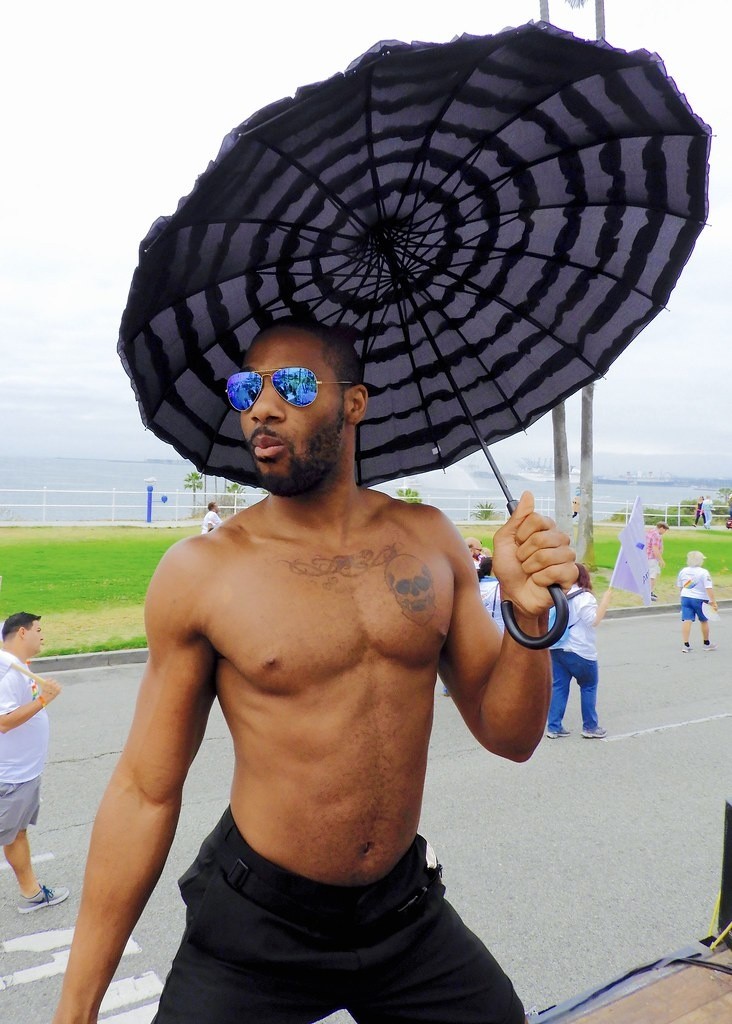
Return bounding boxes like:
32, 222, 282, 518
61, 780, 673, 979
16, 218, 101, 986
38, 696, 46, 707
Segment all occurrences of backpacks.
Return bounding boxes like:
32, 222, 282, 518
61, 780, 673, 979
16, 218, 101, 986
548, 588, 591, 647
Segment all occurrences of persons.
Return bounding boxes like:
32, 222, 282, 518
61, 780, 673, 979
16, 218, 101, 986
645, 521, 669, 600
728, 494, 732, 519
0, 612, 68, 913
571, 489, 580, 517
201, 501, 223, 534
677, 550, 717, 653
692, 496, 706, 527
51, 316, 578, 1024
701, 495, 714, 530
443, 536, 504, 696
547, 564, 612, 738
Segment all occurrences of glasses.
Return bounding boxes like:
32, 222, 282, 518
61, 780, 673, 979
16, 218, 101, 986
225, 367, 357, 412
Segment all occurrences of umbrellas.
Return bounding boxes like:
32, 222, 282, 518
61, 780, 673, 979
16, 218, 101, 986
118, 19, 712, 647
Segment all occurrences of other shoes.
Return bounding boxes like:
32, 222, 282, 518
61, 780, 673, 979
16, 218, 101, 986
651, 593, 656, 599
704, 643, 718, 650
683, 645, 693, 652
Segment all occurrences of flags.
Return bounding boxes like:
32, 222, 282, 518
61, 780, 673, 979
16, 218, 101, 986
605, 498, 651, 605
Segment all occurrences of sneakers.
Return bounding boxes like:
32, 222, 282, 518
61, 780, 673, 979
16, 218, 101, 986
18, 886, 70, 913
546, 730, 570, 738
582, 727, 606, 738
